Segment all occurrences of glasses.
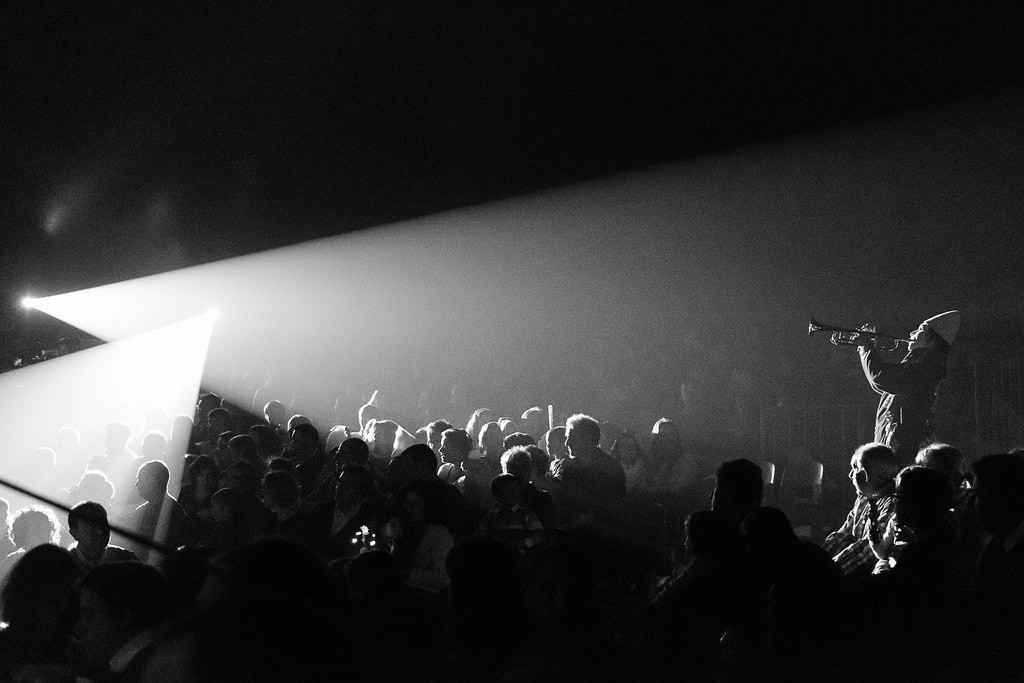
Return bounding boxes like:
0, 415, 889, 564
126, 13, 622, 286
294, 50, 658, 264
884, 487, 903, 502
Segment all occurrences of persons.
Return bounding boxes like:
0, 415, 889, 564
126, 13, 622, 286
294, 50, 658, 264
0, 309, 1023, 683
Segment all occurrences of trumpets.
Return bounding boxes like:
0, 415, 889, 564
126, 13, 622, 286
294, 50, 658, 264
807, 317, 917, 355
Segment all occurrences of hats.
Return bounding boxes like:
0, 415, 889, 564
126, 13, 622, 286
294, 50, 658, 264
924, 309, 962, 346
